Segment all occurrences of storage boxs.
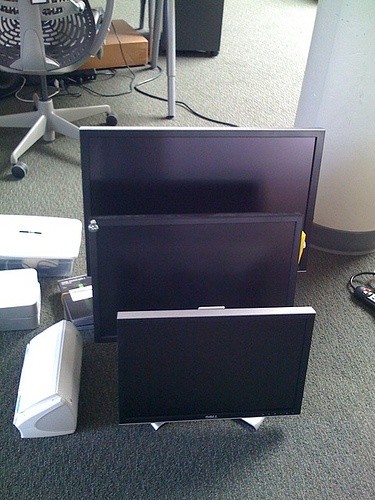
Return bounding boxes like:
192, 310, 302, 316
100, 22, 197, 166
0, 214, 82, 277
81, 18, 147, 70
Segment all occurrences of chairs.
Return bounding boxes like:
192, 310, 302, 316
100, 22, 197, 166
0, 0, 118, 178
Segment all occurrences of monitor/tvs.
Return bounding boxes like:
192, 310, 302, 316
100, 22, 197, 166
117, 305, 316, 424
86, 211, 303, 344
78, 125, 325, 273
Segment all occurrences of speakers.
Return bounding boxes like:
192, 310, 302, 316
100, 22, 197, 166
14, 320, 83, 439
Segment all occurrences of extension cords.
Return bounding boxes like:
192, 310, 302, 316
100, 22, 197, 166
355, 285, 375, 309
47, 67, 97, 88
